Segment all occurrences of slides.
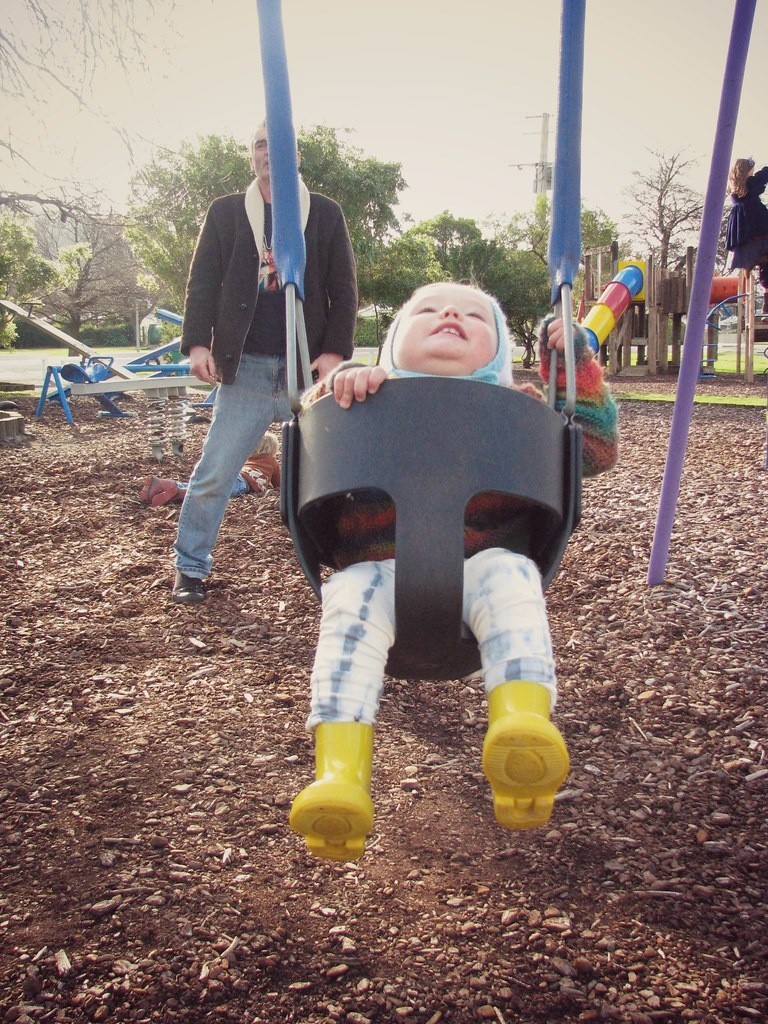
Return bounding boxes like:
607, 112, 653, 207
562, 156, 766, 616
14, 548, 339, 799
580, 265, 644, 357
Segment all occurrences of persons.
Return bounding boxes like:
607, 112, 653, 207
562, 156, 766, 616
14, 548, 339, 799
141, 429, 281, 507
171, 117, 359, 604
723, 158, 768, 315
288, 282, 621, 861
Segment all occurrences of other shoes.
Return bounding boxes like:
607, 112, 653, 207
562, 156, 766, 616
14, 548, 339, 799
171, 569, 207, 600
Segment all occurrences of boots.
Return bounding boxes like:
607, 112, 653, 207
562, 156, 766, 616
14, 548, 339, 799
142, 475, 178, 504
288, 722, 375, 861
483, 680, 570, 831
762, 292, 768, 314
152, 487, 187, 506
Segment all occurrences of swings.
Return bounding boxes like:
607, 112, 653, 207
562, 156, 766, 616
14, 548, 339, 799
257, 0, 590, 683
